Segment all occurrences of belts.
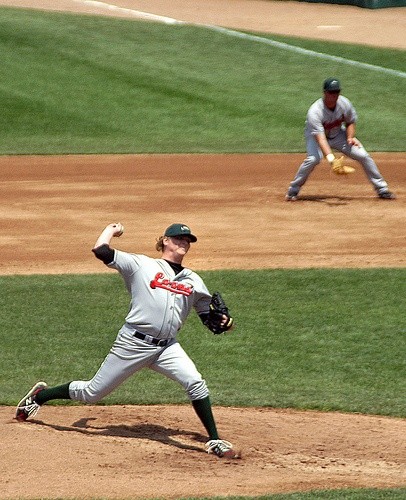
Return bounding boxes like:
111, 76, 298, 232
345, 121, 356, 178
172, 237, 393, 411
134, 332, 170, 346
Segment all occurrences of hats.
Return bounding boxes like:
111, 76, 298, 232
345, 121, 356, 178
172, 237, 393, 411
164, 222, 197, 243
323, 78, 342, 90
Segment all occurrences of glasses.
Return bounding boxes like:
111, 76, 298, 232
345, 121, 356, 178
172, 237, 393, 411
328, 89, 340, 94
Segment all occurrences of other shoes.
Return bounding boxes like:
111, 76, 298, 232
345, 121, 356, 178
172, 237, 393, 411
379, 190, 396, 200
285, 190, 298, 201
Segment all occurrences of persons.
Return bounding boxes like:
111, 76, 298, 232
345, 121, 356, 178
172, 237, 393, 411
284, 78, 395, 201
16, 222, 242, 461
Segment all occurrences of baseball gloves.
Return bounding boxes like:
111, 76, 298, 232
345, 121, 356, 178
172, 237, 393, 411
330, 156, 355, 175
202, 291, 233, 334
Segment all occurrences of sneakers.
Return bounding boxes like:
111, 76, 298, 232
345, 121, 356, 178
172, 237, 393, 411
205, 439, 236, 459
15, 382, 48, 421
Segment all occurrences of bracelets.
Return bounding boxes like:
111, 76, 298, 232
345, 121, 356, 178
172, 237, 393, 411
326, 154, 335, 162
